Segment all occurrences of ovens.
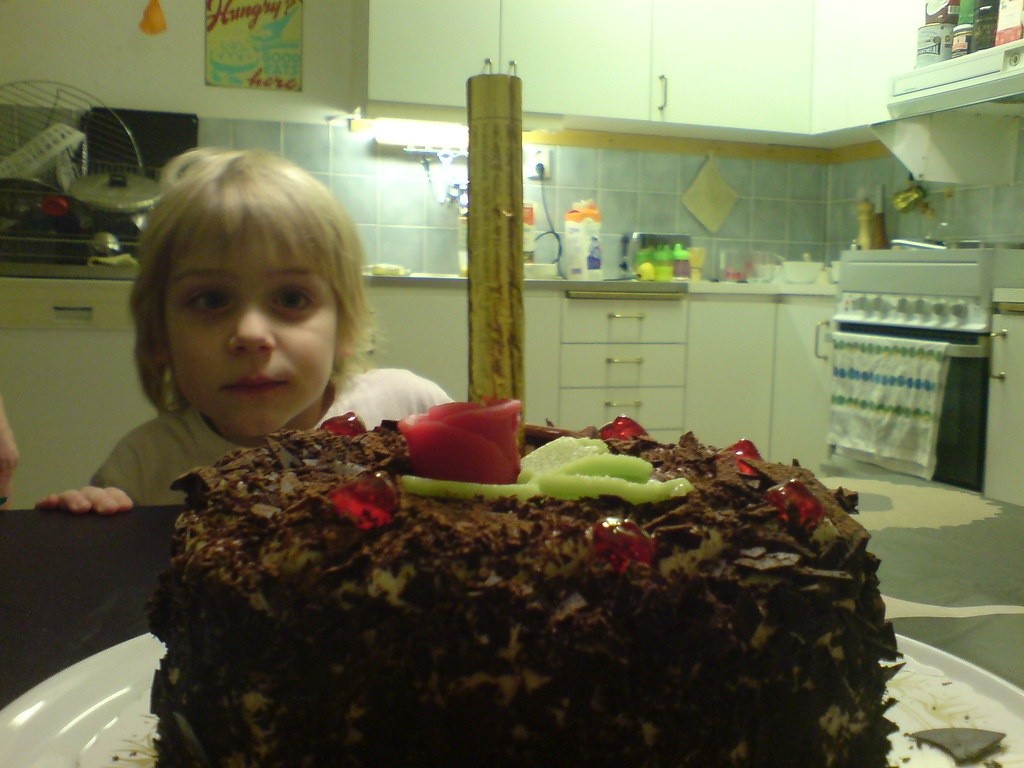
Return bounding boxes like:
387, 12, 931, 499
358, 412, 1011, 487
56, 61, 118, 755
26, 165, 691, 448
832, 323, 988, 493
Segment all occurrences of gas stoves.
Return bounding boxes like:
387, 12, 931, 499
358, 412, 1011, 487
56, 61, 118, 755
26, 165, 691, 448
838, 239, 1023, 332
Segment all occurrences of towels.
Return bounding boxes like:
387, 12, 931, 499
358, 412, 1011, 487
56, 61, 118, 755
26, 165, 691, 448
827, 330, 951, 484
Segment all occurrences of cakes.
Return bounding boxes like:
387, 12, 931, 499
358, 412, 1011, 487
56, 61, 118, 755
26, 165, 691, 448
150, 395, 904, 768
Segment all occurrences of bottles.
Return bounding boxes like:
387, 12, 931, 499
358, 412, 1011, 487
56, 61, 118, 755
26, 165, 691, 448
635, 243, 691, 283
858, 197, 875, 249
916, 0, 1024, 65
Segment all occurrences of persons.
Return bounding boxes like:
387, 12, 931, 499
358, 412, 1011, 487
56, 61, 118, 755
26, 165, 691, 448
32, 148, 451, 518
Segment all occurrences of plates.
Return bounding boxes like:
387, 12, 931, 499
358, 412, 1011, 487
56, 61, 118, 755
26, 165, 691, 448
0, 630, 1024, 768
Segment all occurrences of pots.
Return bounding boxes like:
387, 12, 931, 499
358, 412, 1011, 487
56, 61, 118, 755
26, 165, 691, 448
68, 171, 164, 242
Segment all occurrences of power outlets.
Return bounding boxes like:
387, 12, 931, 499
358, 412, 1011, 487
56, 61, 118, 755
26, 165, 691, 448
527, 152, 551, 176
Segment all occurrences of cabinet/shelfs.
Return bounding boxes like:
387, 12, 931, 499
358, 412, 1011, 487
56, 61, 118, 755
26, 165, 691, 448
352, 1, 1024, 150
982, 288, 1024, 506
0, 275, 834, 508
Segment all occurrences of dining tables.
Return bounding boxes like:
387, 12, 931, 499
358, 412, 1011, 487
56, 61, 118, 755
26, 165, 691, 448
0, 505, 206, 710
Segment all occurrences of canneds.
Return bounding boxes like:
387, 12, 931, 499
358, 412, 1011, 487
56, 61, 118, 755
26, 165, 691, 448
915, 0, 999, 70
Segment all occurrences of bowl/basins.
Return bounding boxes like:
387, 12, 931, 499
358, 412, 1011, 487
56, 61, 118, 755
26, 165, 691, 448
782, 261, 823, 283
523, 261, 556, 278
